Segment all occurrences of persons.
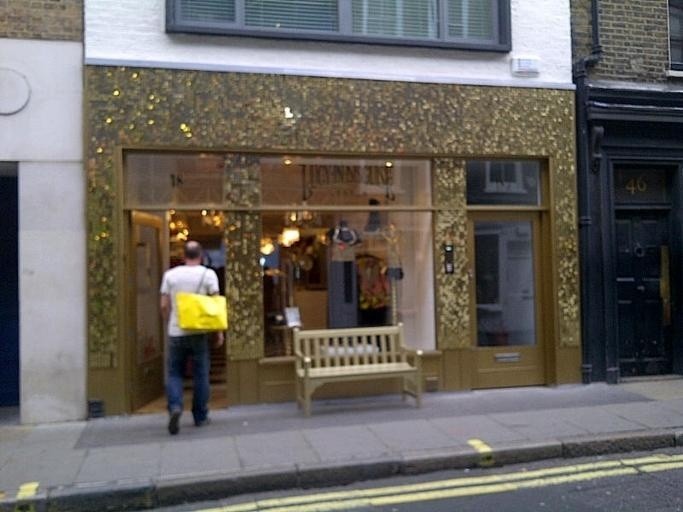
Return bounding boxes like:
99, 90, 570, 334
159, 240, 224, 434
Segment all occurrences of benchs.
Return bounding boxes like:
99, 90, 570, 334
291, 321, 427, 419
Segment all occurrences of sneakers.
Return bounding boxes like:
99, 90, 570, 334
195, 416, 212, 426
168, 408, 183, 435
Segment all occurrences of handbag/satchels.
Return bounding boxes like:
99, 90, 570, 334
176, 292, 229, 332
